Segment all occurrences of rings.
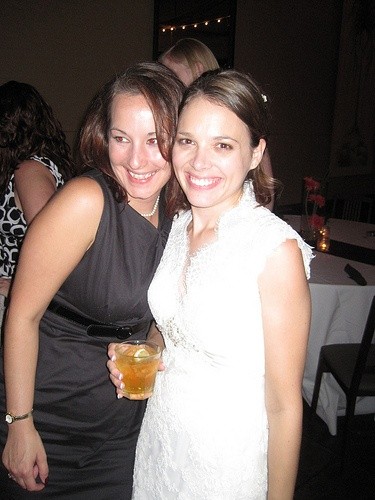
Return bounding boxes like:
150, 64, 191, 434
8, 474, 12, 478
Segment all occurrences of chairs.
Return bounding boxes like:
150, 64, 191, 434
308, 297, 375, 476
331, 195, 374, 223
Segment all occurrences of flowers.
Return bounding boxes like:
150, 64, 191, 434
303, 177, 326, 227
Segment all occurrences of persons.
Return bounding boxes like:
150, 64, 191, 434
106, 70, 315, 500
0, 38, 276, 323
0, 62, 187, 500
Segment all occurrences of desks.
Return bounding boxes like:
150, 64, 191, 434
281, 214, 375, 436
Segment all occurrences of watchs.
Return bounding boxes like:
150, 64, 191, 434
5, 409, 33, 424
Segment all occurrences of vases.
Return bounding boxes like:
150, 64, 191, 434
300, 214, 321, 250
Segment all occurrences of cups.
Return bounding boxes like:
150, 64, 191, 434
316, 225, 330, 252
114, 340, 162, 401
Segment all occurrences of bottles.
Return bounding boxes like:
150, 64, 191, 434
307, 188, 328, 231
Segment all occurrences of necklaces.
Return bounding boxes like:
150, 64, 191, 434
140, 192, 162, 217
